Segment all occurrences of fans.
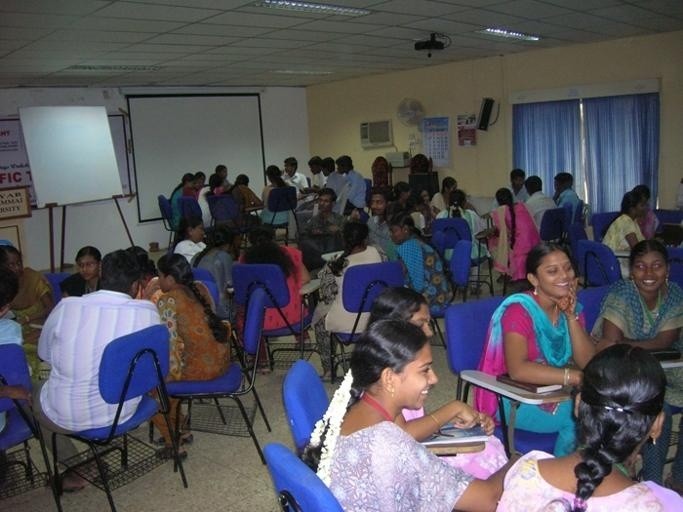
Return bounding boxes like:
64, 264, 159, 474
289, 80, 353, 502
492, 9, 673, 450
394, 96, 429, 135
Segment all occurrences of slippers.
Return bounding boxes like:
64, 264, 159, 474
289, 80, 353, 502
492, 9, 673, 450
48, 473, 85, 492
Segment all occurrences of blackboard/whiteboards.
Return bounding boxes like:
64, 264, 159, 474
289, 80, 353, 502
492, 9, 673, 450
17, 105, 125, 209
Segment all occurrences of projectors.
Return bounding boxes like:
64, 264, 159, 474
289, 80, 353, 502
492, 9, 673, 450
414, 41, 444, 51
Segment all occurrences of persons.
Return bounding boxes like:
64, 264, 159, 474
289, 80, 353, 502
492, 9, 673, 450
0, 246, 231, 492
299, 238, 682, 512
173, 155, 661, 383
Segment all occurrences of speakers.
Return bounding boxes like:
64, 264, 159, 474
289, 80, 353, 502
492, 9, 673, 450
477, 96, 495, 131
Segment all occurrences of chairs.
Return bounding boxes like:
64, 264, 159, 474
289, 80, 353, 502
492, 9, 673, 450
261, 444, 343, 512
162, 291, 267, 473
283, 363, 330, 456
50, 324, 188, 512
2, 376, 66, 506
446, 294, 561, 461
0, 176, 683, 443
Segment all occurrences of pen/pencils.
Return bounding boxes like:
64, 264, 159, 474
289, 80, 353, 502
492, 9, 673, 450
434, 433, 455, 437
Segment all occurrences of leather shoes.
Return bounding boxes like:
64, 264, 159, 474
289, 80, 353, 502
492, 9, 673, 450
154, 431, 194, 445
154, 446, 188, 463
